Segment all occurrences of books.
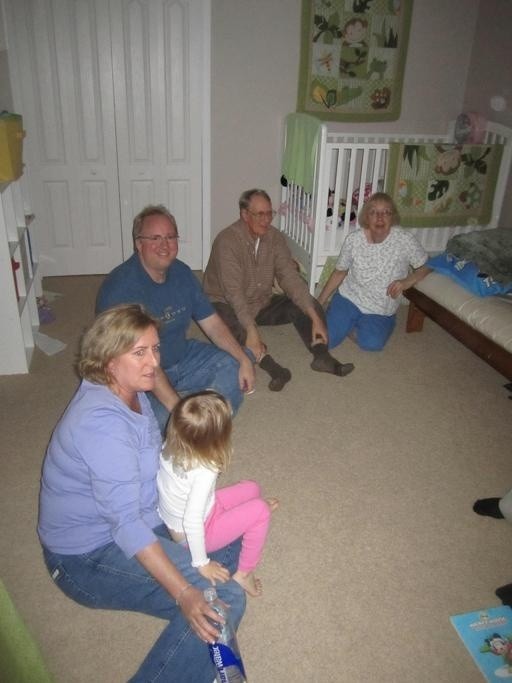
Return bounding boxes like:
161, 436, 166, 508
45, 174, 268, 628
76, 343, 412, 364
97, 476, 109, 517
448, 605, 511, 682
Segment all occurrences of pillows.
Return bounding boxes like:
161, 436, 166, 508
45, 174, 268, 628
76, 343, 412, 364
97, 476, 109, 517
425, 251, 512, 296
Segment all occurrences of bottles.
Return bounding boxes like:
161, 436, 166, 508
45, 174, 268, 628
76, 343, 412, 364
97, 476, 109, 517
200, 585, 251, 683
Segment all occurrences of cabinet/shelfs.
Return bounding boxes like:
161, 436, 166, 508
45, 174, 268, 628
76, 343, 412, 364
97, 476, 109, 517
2, 180, 45, 376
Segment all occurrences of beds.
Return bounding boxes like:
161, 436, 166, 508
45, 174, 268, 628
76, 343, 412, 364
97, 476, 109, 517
402, 263, 512, 381
277, 108, 512, 296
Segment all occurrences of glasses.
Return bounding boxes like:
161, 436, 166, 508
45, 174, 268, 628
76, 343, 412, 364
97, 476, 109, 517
139, 235, 180, 242
370, 209, 394, 216
248, 209, 277, 218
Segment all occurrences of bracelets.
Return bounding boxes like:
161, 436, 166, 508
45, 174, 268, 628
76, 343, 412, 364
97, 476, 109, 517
174, 580, 192, 608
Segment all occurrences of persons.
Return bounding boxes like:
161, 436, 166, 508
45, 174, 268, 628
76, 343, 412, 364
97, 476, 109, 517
155, 389, 278, 598
92, 203, 258, 444
315, 193, 434, 352
36, 302, 248, 682
201, 187, 356, 392
472, 488, 511, 608
